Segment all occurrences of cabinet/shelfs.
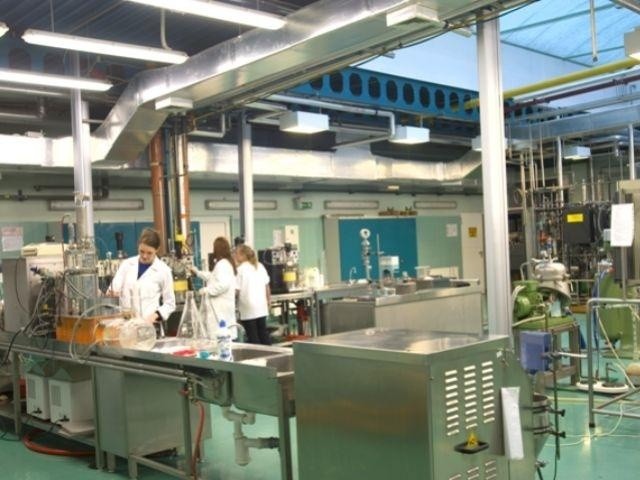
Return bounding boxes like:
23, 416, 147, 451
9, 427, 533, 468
319, 279, 482, 339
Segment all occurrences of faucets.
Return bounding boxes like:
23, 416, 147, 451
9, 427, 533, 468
347, 266, 357, 284
228, 323, 245, 333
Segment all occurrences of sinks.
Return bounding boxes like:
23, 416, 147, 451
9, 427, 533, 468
231, 345, 289, 362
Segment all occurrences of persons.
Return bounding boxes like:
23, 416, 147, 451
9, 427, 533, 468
187, 234, 238, 344
235, 246, 274, 346
229, 235, 250, 268
105, 224, 177, 339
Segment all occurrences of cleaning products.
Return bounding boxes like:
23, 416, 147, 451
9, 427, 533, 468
218, 321, 233, 361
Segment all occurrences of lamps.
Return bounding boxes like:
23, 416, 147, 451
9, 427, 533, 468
563, 146, 591, 160
278, 110, 330, 135
388, 125, 430, 145
0, 0, 290, 94
470, 134, 508, 153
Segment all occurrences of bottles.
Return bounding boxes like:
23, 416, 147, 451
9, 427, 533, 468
175, 290, 232, 362
